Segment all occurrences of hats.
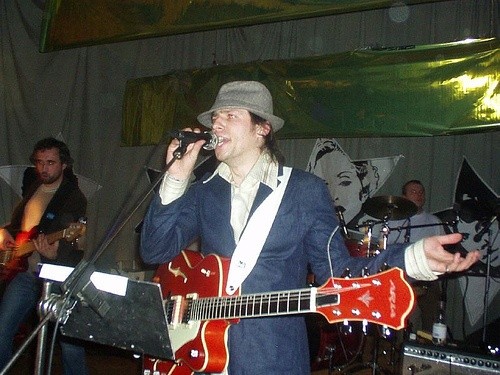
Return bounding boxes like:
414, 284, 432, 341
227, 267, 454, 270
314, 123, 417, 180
197, 80, 285, 133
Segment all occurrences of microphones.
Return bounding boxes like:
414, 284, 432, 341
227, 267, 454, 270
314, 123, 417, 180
169, 129, 219, 151
473, 215, 498, 242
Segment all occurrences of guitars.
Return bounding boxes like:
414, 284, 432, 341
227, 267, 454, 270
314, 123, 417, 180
141, 249, 417, 375
0, 217, 87, 279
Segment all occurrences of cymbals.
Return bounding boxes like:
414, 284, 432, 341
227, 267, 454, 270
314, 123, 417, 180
363, 196, 417, 221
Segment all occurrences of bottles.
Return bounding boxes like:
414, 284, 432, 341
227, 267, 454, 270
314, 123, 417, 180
432, 299, 447, 348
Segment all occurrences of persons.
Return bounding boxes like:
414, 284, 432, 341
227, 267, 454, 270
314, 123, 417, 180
138, 78, 482, 375
369, 179, 449, 333
1, 137, 91, 375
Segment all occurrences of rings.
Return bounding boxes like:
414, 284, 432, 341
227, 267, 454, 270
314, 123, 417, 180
446, 264, 451, 272
